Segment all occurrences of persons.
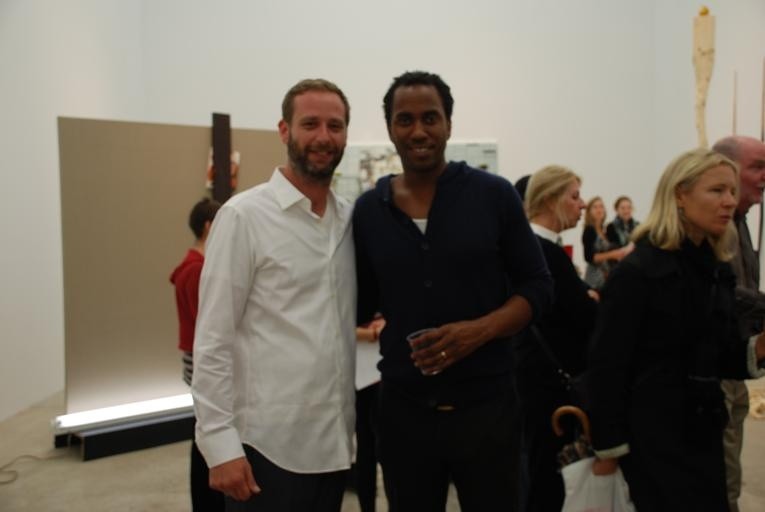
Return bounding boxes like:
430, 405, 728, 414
580, 196, 633, 288
357, 301, 394, 512
513, 174, 531, 200
707, 135, 765, 294
583, 148, 765, 511
168, 197, 223, 512
523, 163, 601, 377
180, 77, 356, 511
606, 196, 643, 246
352, 70, 553, 512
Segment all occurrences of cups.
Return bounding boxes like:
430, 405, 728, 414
408, 327, 443, 377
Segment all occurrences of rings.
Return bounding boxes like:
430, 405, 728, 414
440, 351, 448, 359
